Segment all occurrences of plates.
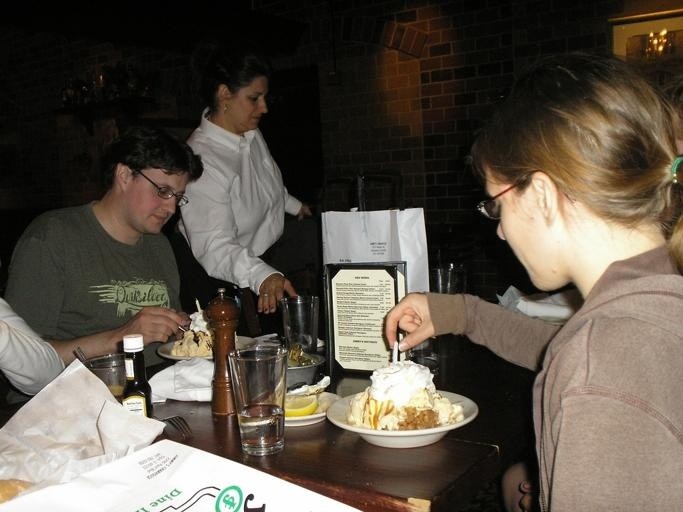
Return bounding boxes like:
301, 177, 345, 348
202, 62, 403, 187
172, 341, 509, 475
157, 336, 259, 361
283, 389, 483, 449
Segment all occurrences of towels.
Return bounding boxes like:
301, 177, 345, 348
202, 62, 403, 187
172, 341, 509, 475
147, 358, 214, 404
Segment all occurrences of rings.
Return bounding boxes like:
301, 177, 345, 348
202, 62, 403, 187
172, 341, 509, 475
261, 292, 268, 297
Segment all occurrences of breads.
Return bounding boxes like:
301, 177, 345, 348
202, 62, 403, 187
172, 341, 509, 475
0, 476, 33, 502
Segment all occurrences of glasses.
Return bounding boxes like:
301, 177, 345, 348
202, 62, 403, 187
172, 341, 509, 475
128, 165, 189, 207
476, 183, 520, 220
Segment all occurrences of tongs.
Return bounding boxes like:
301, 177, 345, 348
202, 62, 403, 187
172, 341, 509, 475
350, 358, 461, 424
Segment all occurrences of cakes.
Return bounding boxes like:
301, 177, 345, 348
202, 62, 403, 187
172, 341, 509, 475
347, 361, 466, 433
171, 310, 215, 358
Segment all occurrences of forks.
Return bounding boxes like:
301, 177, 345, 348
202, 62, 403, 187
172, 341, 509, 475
152, 416, 194, 443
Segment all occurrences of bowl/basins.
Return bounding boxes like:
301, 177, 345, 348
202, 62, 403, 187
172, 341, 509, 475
286, 354, 326, 390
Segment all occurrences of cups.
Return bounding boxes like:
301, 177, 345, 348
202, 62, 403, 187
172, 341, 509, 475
228, 295, 320, 457
84, 351, 126, 398
432, 266, 466, 295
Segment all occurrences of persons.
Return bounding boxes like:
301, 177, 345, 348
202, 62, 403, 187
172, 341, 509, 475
378, 46, 683, 512
0, 298, 66, 401
3, 132, 206, 406
168, 55, 315, 338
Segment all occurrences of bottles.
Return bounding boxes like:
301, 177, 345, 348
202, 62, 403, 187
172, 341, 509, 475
60, 72, 152, 107
120, 333, 153, 419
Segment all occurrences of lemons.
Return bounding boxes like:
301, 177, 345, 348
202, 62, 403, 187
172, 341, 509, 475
283, 394, 318, 420
